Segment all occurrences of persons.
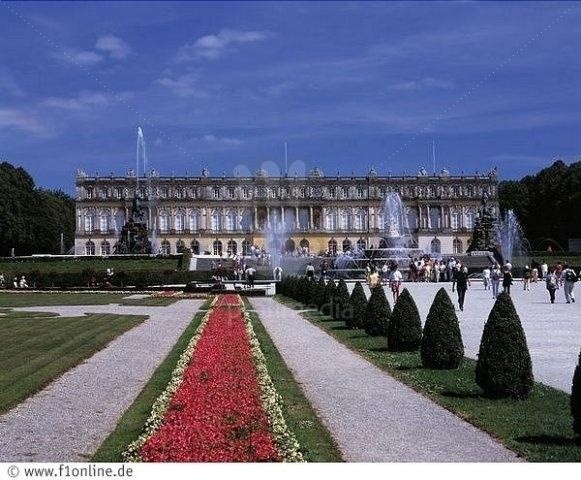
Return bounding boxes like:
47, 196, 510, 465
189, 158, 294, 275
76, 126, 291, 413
0, 271, 28, 289
83, 265, 114, 291
